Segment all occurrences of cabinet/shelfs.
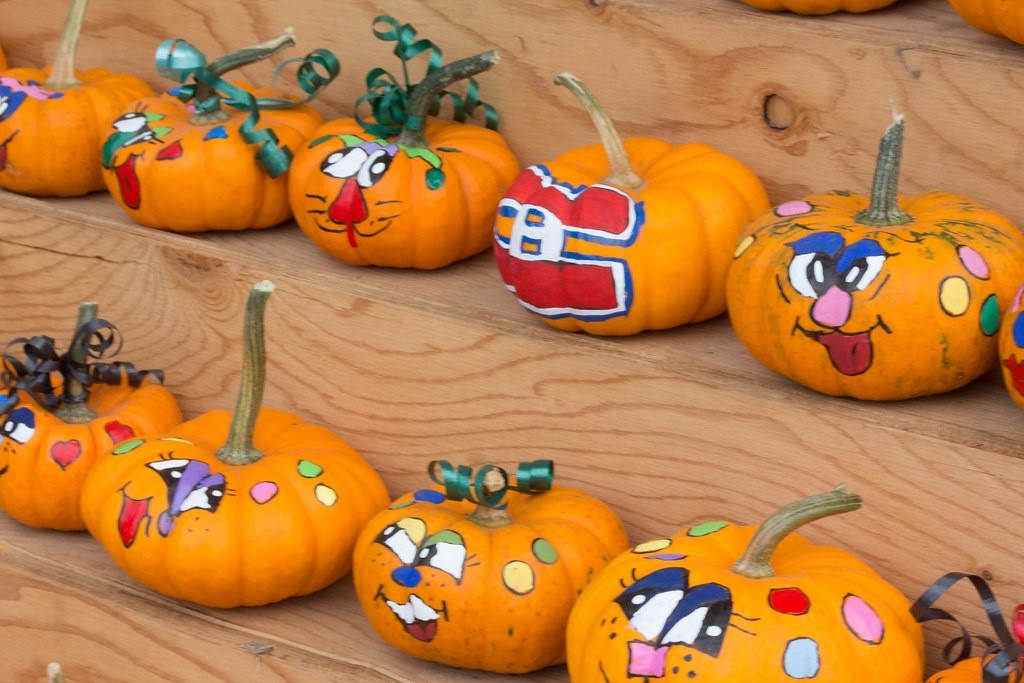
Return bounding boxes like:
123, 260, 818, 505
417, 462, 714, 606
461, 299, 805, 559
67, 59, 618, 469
0, 0, 1024, 683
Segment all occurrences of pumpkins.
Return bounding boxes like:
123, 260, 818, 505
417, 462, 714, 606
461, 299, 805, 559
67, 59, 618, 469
947, 0, 1024, 47
738, 0, 898, 15
